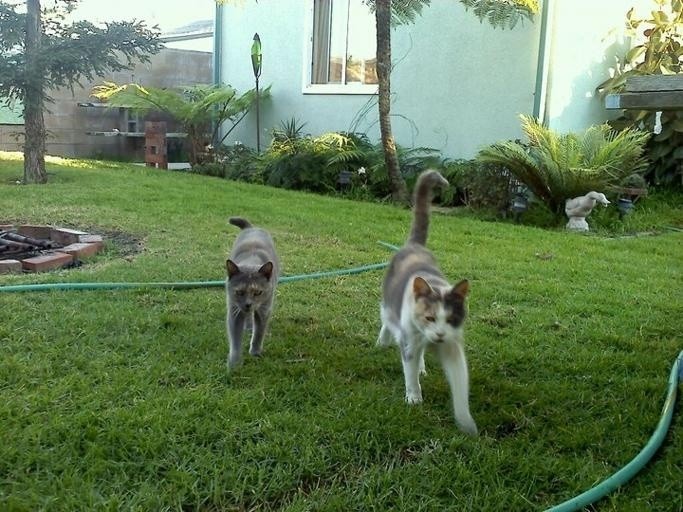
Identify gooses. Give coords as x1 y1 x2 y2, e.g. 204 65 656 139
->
564 191 611 218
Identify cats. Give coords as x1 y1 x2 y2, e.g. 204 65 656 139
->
376 168 479 437
220 215 279 373
